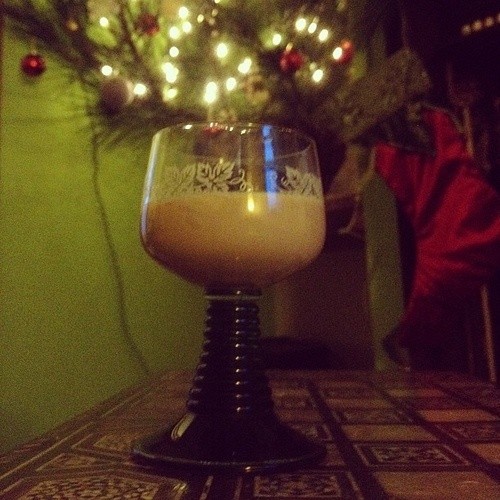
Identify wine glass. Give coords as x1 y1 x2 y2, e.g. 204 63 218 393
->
133 121 326 471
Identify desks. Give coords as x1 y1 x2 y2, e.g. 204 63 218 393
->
0 367 500 500
